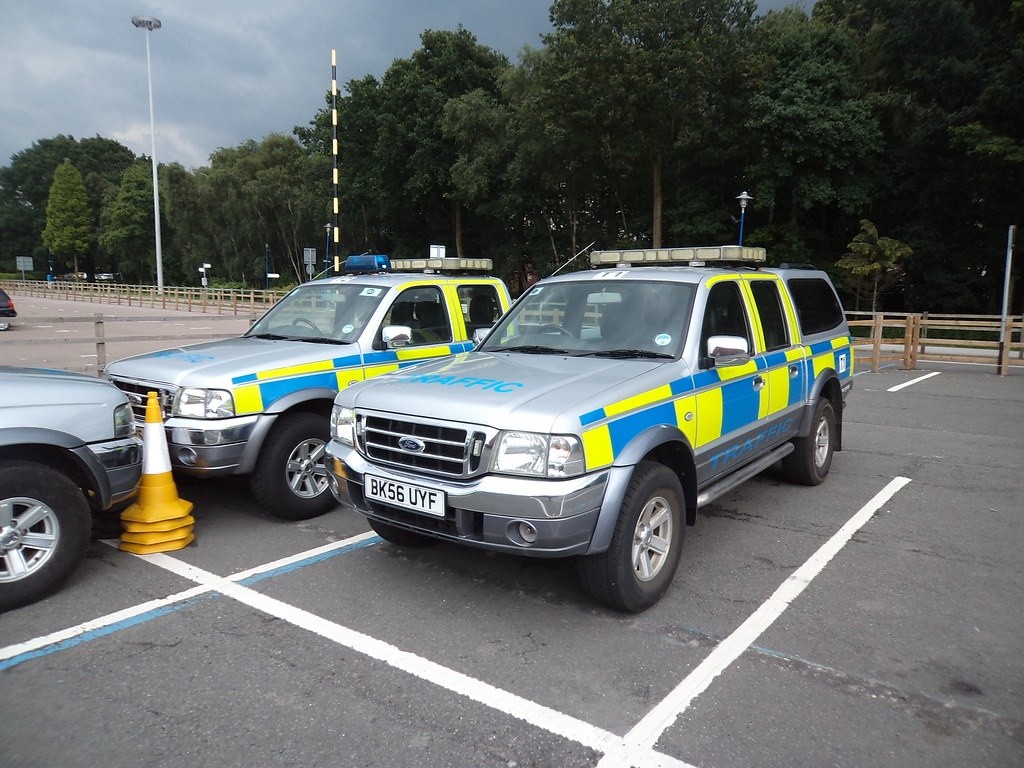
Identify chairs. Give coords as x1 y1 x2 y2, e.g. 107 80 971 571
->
602 303 718 354
470 299 493 323
390 299 445 344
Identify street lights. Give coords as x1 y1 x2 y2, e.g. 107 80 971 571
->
132 14 165 297
735 191 755 246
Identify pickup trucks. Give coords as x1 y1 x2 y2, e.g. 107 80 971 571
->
106 248 602 522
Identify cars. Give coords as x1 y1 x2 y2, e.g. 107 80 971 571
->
0 288 16 331
63 271 123 286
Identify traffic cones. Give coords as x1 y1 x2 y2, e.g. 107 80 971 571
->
119 392 197 554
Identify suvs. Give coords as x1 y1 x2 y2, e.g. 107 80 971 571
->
0 364 143 614
323 243 857 615
